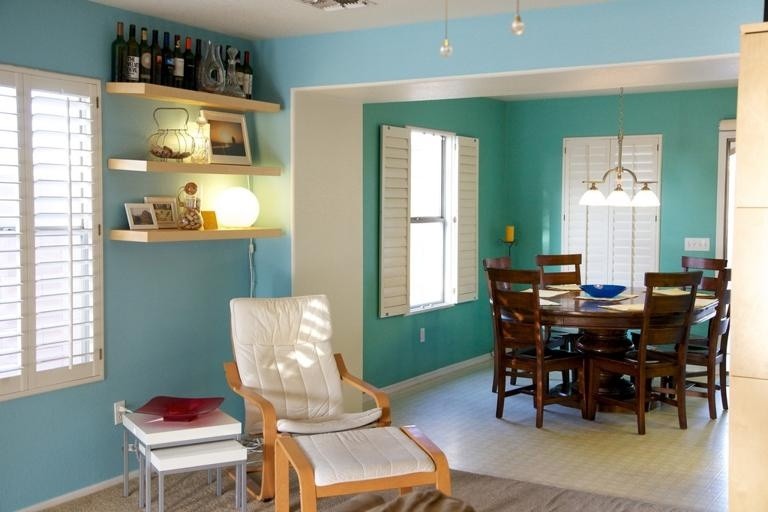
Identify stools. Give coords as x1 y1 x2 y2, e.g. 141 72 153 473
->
135 441 248 512
272 423 453 512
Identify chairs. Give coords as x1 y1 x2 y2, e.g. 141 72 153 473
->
630 256 732 420
482 254 588 429
222 291 393 504
588 271 703 434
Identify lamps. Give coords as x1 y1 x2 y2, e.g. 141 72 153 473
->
214 185 260 229
578 84 661 210
510 1 526 39
440 0 451 57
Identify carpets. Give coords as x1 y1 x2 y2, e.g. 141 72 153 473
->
39 431 707 512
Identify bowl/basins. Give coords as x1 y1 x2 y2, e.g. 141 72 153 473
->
579 284 626 298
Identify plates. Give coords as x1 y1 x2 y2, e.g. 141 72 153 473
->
131 395 225 422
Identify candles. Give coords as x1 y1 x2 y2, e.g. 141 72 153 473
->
504 225 514 243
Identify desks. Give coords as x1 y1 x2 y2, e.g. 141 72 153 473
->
521 284 719 414
116 411 252 512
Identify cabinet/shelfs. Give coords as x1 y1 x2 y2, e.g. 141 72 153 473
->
102 78 289 246
727 18 768 511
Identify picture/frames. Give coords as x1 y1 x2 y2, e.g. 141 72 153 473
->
197 110 254 167
143 196 182 228
123 202 160 232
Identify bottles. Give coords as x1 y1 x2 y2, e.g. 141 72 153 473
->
110 22 253 99
175 180 202 230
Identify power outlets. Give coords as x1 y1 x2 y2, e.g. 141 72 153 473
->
112 400 128 427
